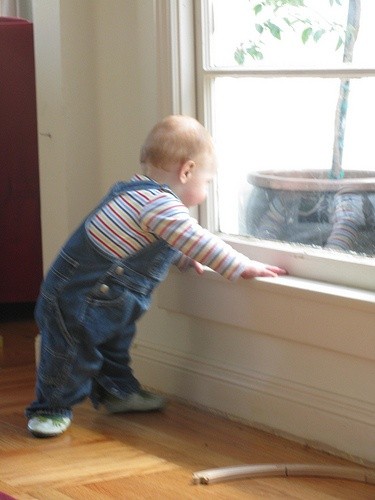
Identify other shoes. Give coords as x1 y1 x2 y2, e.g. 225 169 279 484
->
28 415 70 435
109 390 164 412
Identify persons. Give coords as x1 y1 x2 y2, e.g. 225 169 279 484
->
24 115 287 436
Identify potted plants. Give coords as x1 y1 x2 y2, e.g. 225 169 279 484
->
234 0 375 258
